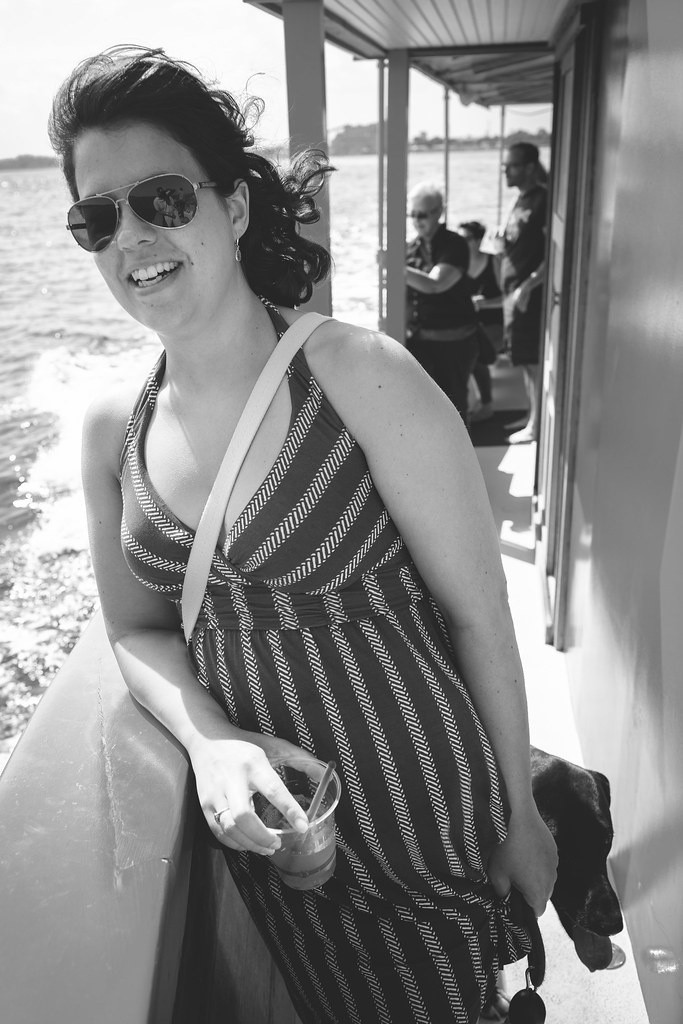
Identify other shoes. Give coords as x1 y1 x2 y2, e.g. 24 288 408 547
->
478 401 492 418
502 413 530 432
505 425 537 446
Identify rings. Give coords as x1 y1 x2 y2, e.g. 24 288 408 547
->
214 808 228 824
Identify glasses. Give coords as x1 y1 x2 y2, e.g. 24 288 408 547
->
500 162 526 173
408 205 442 219
67 174 221 254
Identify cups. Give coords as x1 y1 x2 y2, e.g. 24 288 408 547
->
250 757 344 892
471 295 485 311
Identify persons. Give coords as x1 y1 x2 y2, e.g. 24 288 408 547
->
377 142 550 445
48 44 560 1024
